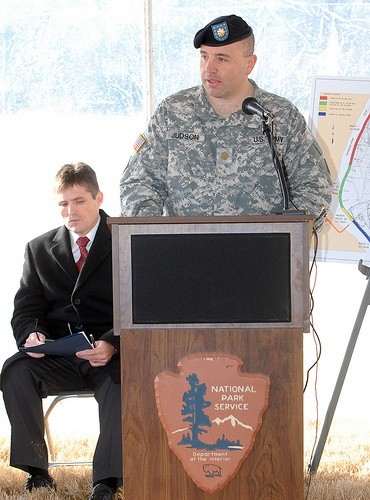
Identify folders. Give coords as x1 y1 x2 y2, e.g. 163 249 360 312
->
19 332 94 357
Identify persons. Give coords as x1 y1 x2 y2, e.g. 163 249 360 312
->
0 162 123 500
120 15 332 232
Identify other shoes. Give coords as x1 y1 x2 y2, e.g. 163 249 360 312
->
89 484 115 500
26 474 57 494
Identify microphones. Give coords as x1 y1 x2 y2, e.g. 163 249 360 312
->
242 97 274 121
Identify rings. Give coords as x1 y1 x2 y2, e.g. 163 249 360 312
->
94 360 96 363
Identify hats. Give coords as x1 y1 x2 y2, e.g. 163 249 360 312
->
194 14 252 49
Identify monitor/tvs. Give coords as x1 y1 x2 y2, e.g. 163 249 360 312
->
117 222 302 328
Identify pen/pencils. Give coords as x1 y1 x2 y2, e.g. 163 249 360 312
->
90 334 96 348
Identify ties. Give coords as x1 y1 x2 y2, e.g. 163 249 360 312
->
75 236 90 272
